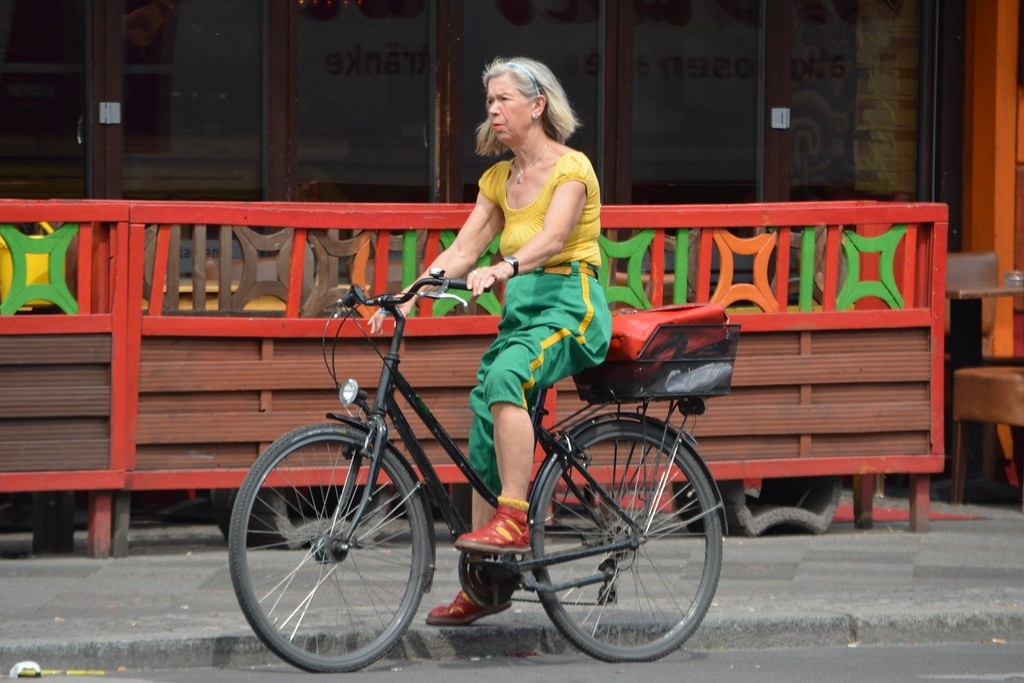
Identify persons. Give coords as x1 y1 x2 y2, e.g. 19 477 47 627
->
367 56 610 625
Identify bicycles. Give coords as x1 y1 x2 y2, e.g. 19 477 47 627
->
228 266 744 676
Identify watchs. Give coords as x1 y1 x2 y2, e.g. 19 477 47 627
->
504 256 518 278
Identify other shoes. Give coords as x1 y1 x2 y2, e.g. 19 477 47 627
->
454 498 532 553
424 570 512 627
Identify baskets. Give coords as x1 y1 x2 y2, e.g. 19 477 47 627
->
572 323 741 397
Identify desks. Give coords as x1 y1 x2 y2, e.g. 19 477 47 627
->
945 279 1024 484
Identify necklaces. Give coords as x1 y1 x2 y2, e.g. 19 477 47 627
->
517 139 550 183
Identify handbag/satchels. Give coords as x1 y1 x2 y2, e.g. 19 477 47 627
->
602 300 732 383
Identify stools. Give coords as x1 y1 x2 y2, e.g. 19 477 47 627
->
948 367 1023 506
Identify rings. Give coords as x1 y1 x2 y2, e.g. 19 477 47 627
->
473 270 478 275
380 309 389 316
491 274 497 282
